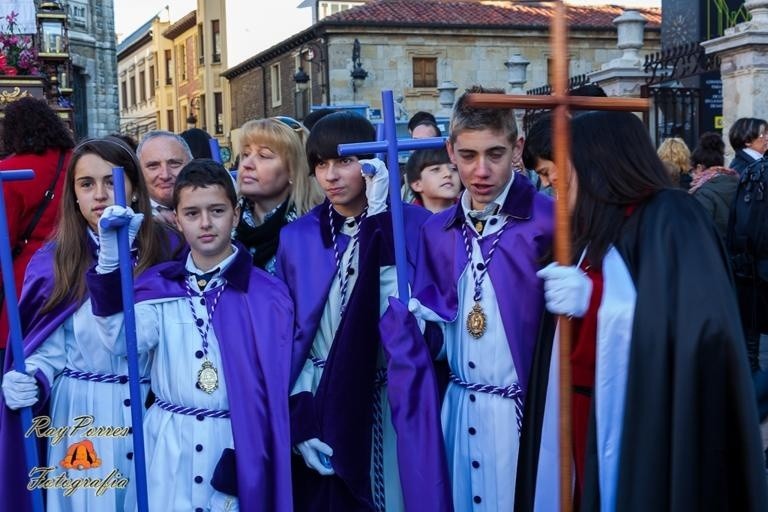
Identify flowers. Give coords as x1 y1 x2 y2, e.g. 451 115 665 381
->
1 9 39 77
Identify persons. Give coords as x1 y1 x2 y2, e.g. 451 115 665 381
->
0 97 766 426
515 105 767 512
1 136 192 511
379 85 559 511
273 111 436 511
88 159 297 512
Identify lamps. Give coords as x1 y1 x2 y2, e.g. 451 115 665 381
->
185 94 204 130
351 38 369 94
293 40 324 92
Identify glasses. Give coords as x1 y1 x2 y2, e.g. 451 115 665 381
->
753 133 768 138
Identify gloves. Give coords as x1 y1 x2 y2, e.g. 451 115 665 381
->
95 205 145 274
296 437 336 478
357 155 390 220
535 261 593 318
408 298 426 335
2 361 43 414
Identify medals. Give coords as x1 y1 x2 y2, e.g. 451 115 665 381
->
195 360 221 395
463 302 488 339
